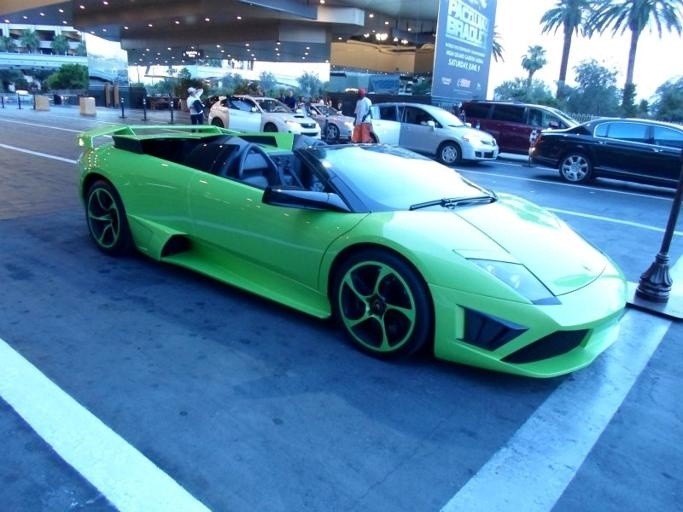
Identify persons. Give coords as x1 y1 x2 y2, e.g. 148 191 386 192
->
185 85 206 134
350 87 372 144
276 89 344 114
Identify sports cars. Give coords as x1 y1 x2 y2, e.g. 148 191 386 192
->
71 122 630 381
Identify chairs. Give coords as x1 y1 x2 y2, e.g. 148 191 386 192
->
221 138 283 188
290 135 328 188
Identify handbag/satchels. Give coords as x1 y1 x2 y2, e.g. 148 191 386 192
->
192 100 204 113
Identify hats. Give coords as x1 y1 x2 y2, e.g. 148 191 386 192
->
188 87 195 94
358 88 366 97
